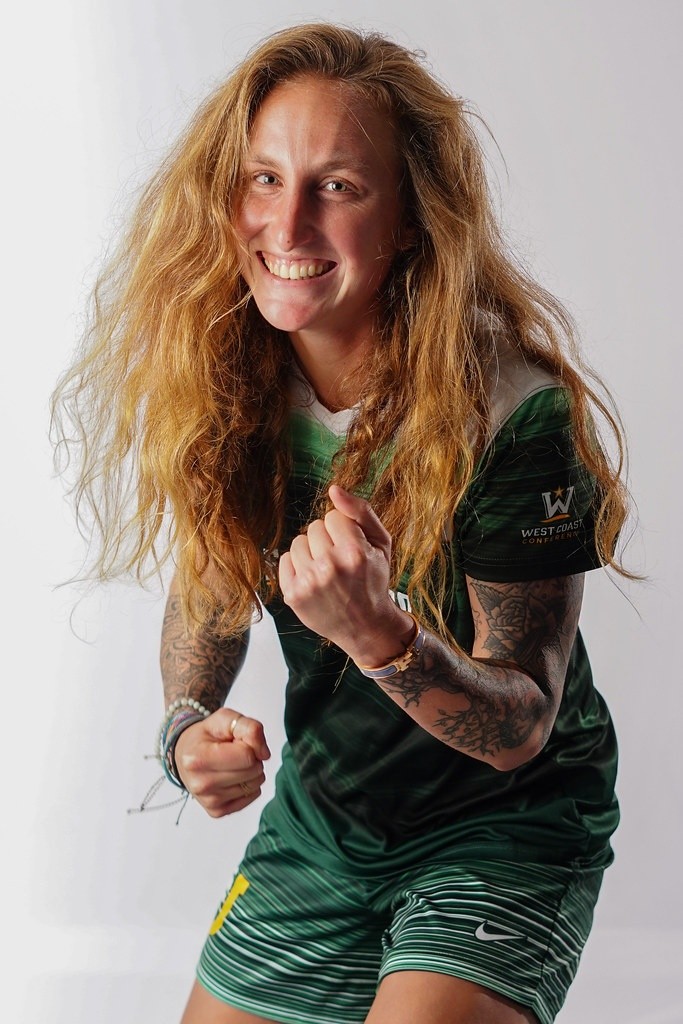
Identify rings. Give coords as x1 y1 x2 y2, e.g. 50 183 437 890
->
230 713 243 734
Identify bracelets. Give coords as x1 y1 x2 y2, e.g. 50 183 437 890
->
358 610 426 678
127 697 212 825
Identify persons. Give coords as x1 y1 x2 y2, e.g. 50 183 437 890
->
47 25 639 1024
